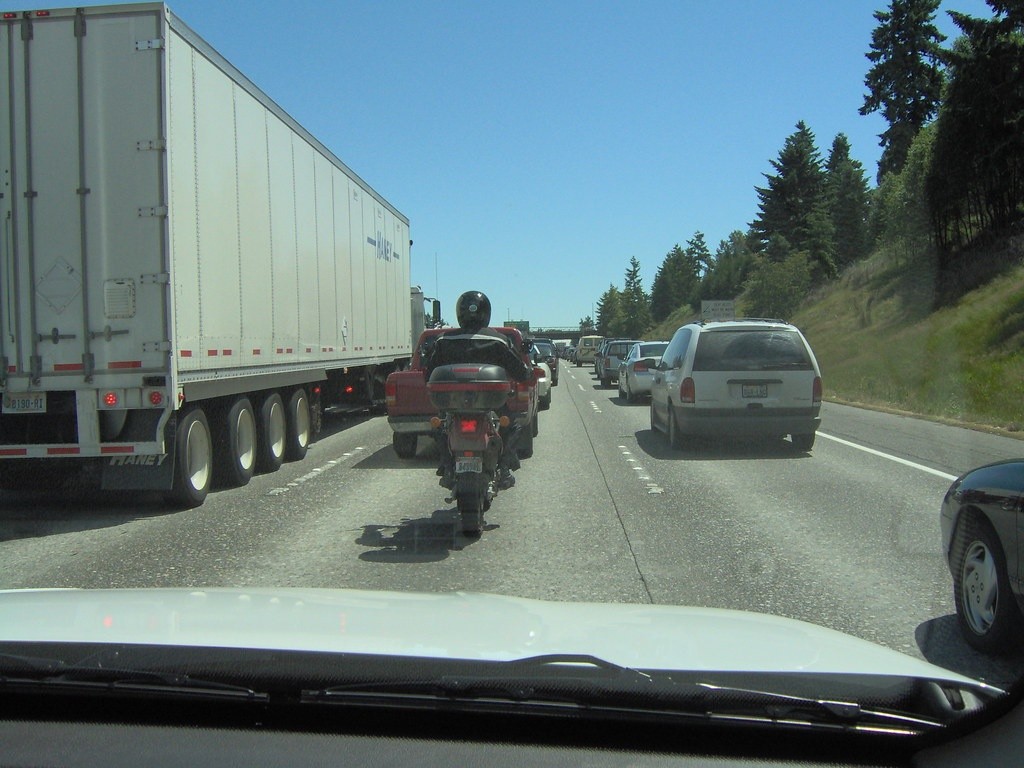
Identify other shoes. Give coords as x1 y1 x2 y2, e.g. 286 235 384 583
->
436 462 446 477
496 463 510 479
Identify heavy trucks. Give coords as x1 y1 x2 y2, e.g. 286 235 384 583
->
0 1 441 515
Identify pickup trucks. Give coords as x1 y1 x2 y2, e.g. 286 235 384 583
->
385 327 544 460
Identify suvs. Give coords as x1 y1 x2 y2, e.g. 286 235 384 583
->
576 335 604 367
649 314 823 448
525 338 561 388
594 338 646 388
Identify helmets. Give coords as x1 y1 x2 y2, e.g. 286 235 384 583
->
455 291 490 330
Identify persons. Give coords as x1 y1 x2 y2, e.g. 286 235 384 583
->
426 291 526 489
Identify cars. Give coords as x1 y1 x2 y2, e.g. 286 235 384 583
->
554 336 578 364
616 339 671 402
529 344 554 411
940 457 1023 657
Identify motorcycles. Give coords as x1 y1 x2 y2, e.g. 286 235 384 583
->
428 359 531 536
